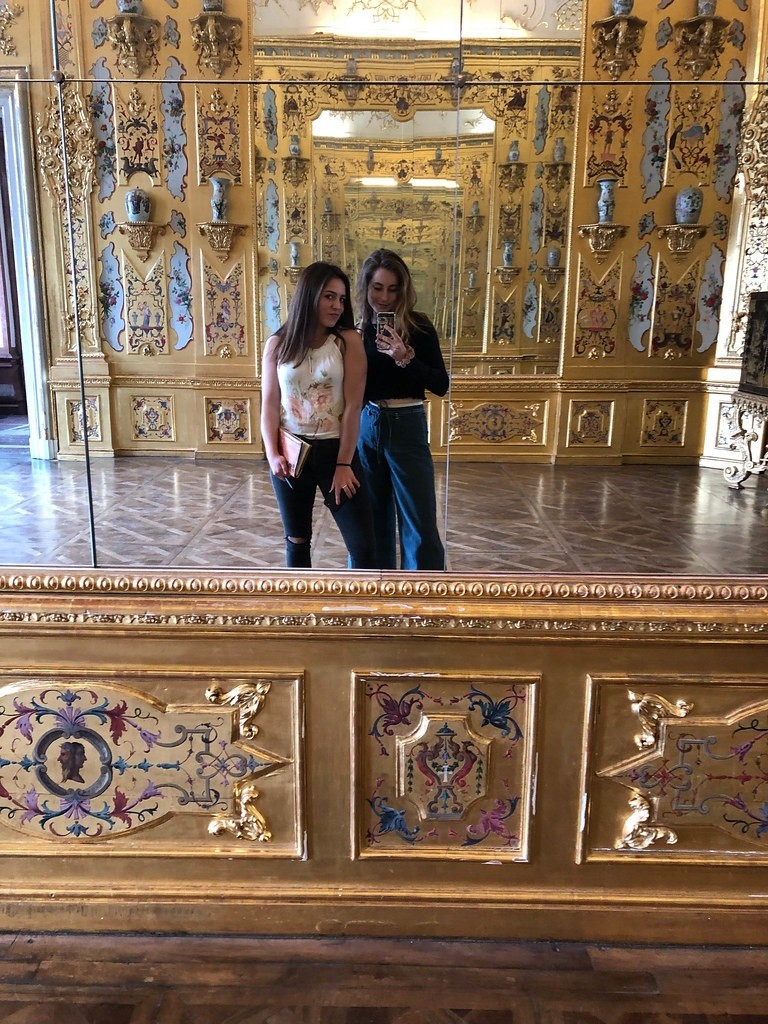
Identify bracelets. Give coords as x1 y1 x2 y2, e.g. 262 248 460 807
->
335 463 352 466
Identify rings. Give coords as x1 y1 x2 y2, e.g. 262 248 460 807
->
342 484 347 488
387 345 390 349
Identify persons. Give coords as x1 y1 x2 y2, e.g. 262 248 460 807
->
357 249 449 570
258 260 377 569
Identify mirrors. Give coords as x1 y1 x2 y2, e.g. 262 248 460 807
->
247 0 588 379
0 0 768 603
312 109 497 354
343 175 465 347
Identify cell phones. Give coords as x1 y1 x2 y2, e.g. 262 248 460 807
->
376 312 395 349
324 482 358 512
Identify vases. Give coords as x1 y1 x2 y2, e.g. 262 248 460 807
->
596 177 619 222
467 271 476 287
324 198 333 213
289 242 301 266
288 134 301 156
471 201 479 215
434 145 443 160
554 136 567 162
503 241 515 266
209 177 232 222
509 140 520 161
547 248 561 267
346 57 357 74
450 48 464 74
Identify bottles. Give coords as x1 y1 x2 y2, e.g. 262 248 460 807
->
125 186 151 221
546 244 561 267
675 183 703 224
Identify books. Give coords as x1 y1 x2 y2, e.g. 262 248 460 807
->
278 427 312 478
